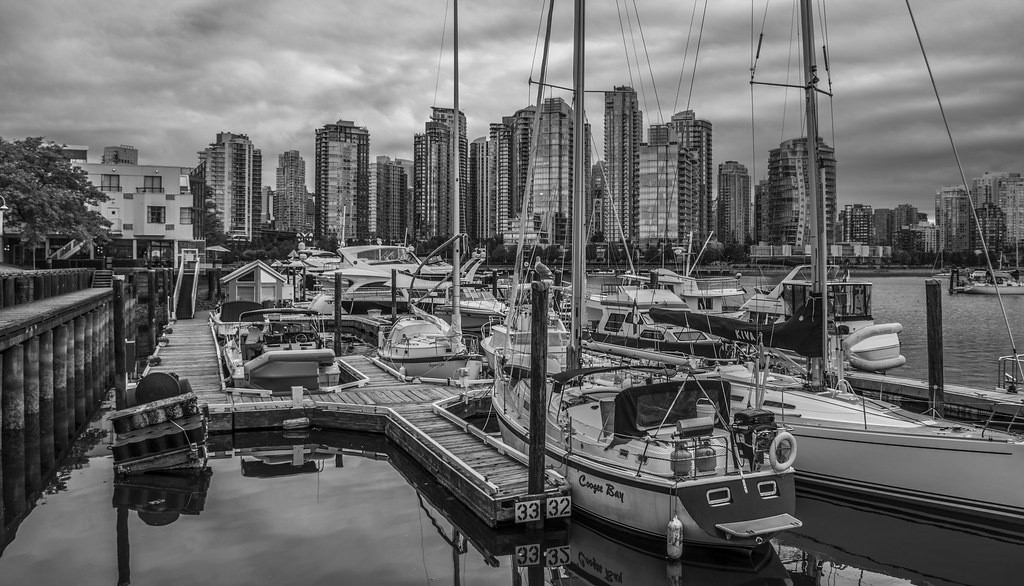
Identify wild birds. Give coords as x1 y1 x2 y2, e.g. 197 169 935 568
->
533 255 556 283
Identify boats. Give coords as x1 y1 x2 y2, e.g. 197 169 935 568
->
947 268 1024 295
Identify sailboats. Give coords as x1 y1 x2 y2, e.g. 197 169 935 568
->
209 0 1024 581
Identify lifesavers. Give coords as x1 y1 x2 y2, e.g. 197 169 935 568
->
767 430 799 474
